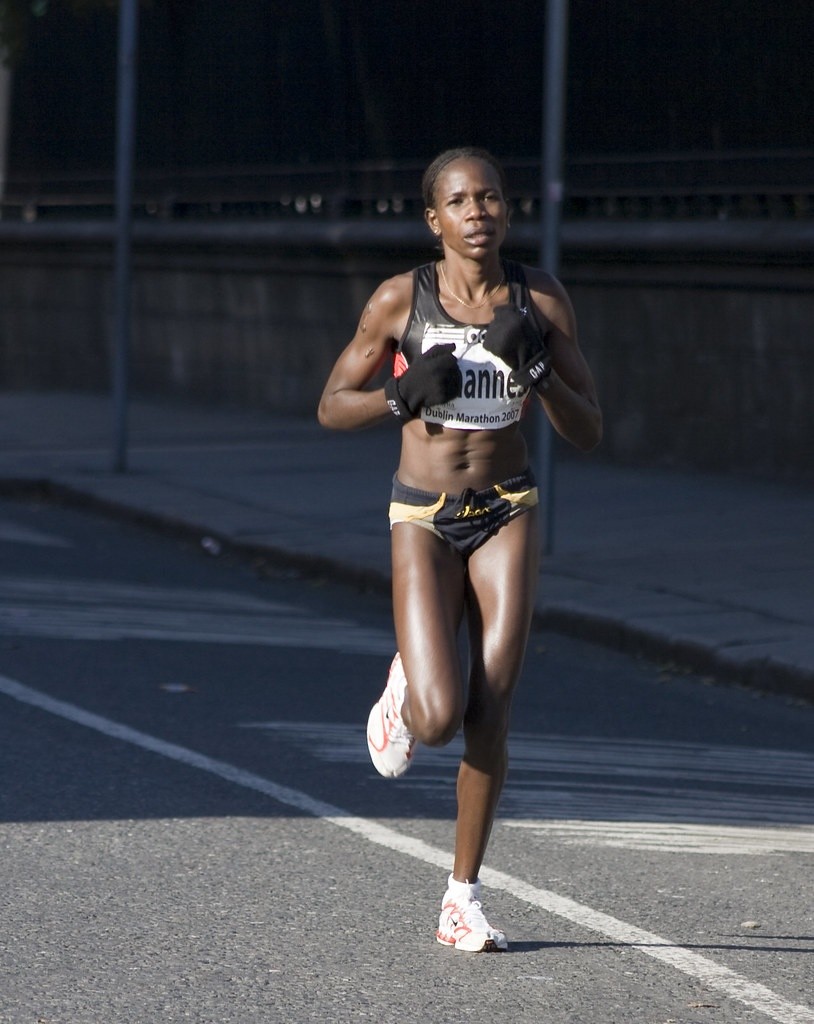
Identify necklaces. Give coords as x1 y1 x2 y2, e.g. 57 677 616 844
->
439 260 504 309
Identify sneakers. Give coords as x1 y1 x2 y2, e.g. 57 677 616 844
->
437 891 508 952
367 651 417 778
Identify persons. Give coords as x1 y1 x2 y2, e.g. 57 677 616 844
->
319 146 601 952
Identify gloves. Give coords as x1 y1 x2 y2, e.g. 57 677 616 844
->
482 304 552 388
384 343 463 425
